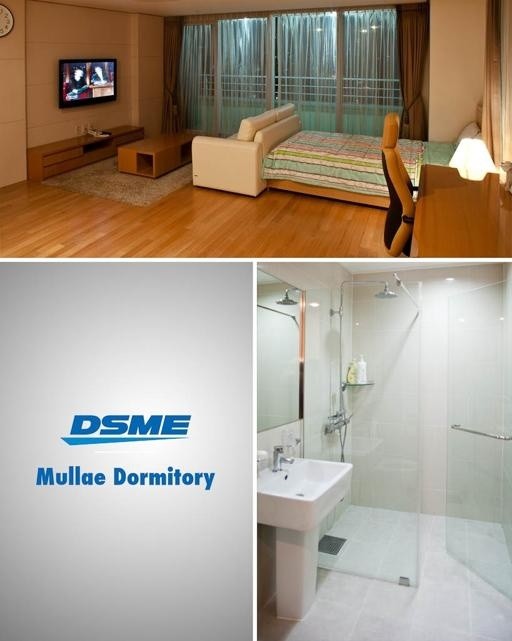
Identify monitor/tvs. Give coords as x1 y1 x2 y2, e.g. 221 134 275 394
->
59 59 117 108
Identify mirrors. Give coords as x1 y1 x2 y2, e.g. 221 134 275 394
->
257 268 305 433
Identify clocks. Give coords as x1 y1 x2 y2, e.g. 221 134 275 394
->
0 5 14 37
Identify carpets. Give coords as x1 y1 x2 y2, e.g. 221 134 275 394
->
45 154 193 206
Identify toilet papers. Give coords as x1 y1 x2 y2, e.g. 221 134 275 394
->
357 355 367 383
347 358 358 383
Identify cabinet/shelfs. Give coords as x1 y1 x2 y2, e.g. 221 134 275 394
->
27 123 145 181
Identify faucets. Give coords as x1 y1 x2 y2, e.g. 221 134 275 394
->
272 445 294 472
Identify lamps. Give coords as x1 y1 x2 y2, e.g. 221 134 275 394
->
447 135 512 192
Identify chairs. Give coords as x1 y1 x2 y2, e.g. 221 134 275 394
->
373 109 421 257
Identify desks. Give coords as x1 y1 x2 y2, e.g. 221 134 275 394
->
410 161 512 257
116 129 205 178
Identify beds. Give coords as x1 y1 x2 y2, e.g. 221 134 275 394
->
264 119 481 210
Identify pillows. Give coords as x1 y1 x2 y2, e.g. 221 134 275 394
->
454 122 480 148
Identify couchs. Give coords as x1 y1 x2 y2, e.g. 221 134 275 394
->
194 101 301 197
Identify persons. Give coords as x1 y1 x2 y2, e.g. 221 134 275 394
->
90 65 110 86
66 69 88 94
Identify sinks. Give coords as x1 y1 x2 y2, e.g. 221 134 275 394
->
257 457 352 503
